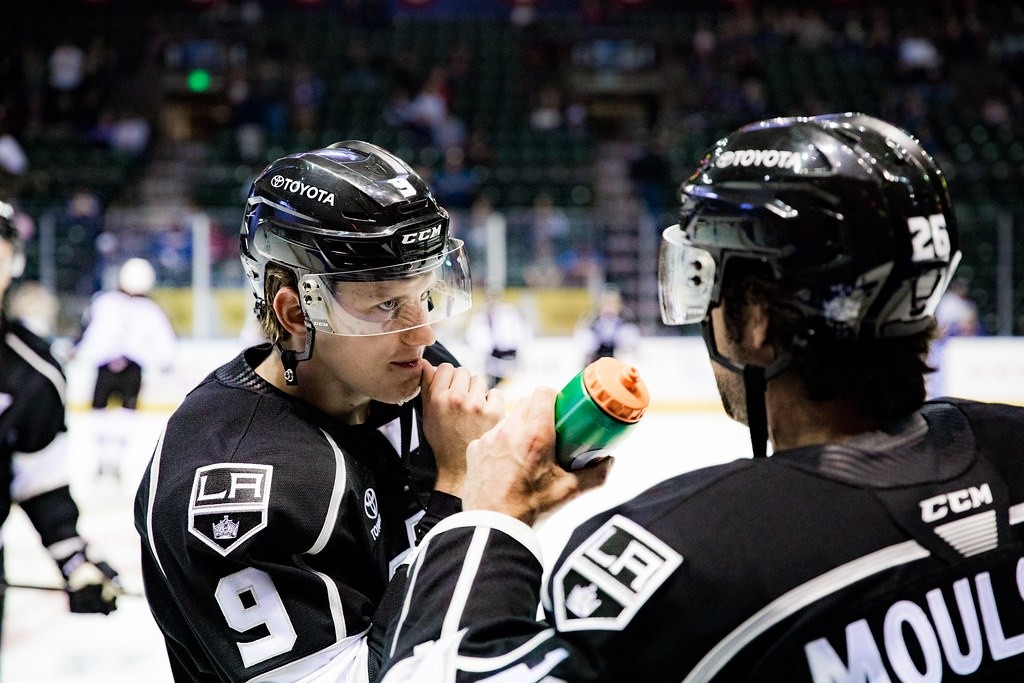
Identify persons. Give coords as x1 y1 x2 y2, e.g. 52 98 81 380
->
374 113 1024 683
0 188 125 650
133 140 506 683
2 0 1024 417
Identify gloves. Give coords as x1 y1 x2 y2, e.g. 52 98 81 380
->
54 545 124 615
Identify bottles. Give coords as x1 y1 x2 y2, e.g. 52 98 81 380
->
554 357 650 473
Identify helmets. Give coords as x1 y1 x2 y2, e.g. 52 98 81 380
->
678 110 959 345
237 139 451 331
